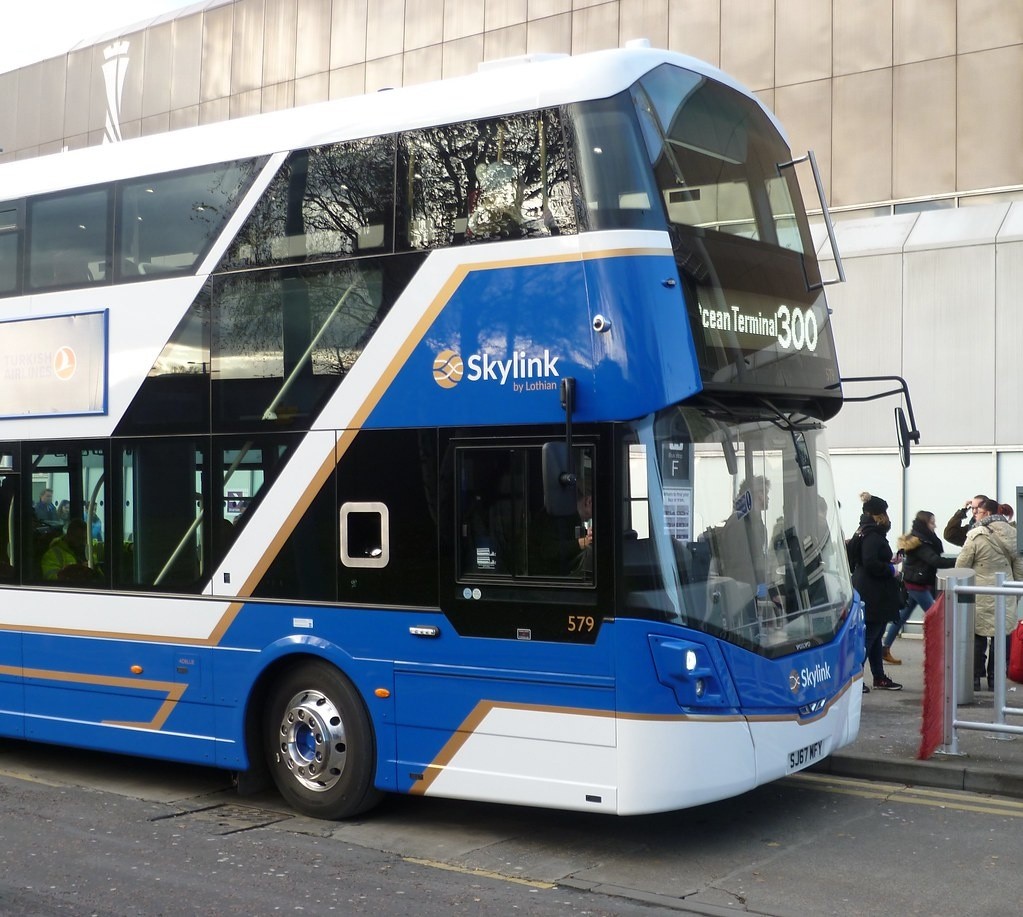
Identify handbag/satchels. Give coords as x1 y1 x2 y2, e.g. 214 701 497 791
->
896 578 909 611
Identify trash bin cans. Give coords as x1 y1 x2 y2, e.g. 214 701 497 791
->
935 567 977 705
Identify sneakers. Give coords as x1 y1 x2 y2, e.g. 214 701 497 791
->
880 645 901 666
871 675 905 693
862 683 869 693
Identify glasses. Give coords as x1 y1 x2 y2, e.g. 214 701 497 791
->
969 506 980 512
974 510 988 516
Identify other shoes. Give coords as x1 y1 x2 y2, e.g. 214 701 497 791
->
971 672 984 690
986 671 996 691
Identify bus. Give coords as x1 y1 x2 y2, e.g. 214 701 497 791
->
0 39 921 822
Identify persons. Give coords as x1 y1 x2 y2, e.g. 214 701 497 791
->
842 491 903 694
943 494 991 678
881 509 957 665
0 433 841 657
953 500 1023 693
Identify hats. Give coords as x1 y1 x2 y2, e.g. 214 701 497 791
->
860 492 889 515
